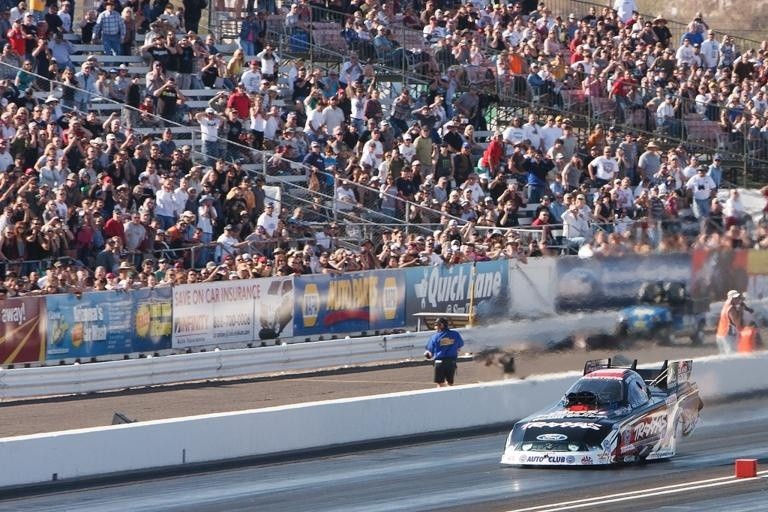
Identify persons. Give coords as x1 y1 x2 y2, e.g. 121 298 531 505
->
423 317 464 388
1 1 768 300
715 289 745 356
735 294 757 328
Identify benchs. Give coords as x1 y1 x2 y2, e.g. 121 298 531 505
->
413 312 475 333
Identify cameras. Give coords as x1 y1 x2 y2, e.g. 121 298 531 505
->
219 267 226 270
739 303 753 313
75 137 80 141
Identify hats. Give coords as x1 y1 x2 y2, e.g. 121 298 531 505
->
67 173 78 181
180 210 196 222
199 195 213 203
727 290 739 298
118 262 130 270
89 133 115 146
435 318 447 324
25 168 35 174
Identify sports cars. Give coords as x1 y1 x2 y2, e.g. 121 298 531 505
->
500 356 704 469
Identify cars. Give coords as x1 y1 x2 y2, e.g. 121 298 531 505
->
613 278 710 346
